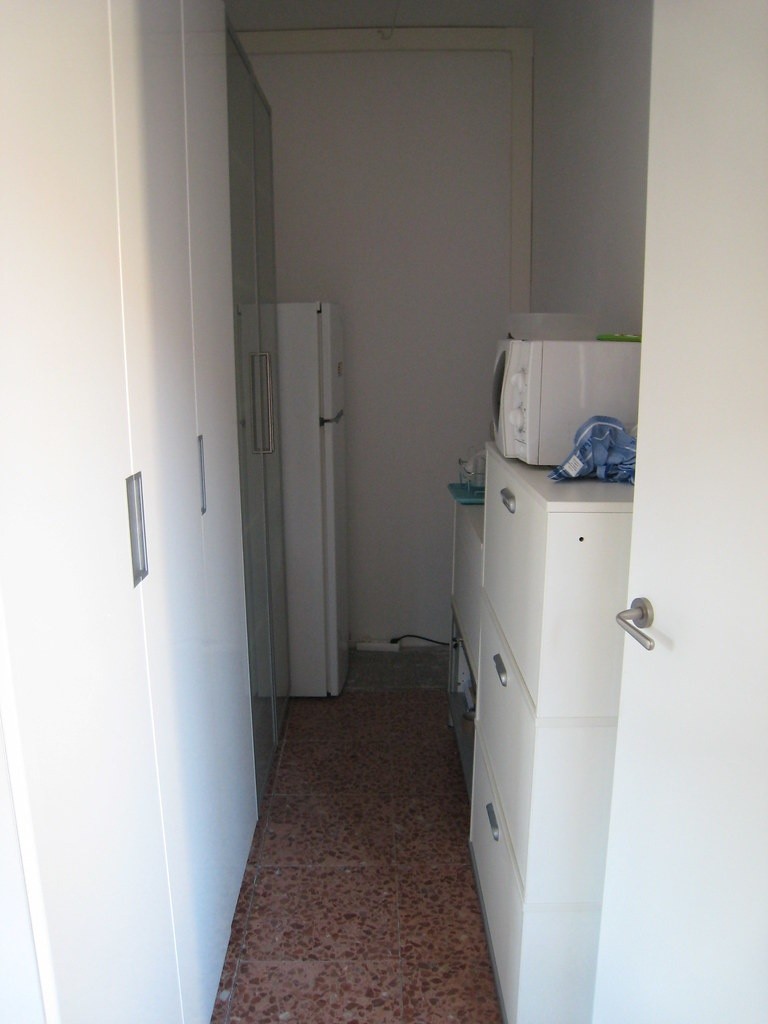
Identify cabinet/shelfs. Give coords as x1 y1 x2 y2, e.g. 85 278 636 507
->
0 0 281 1024
469 439 636 1024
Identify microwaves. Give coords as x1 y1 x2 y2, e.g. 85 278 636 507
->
489 337 642 465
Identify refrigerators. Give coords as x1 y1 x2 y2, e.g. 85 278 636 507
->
278 301 350 696
